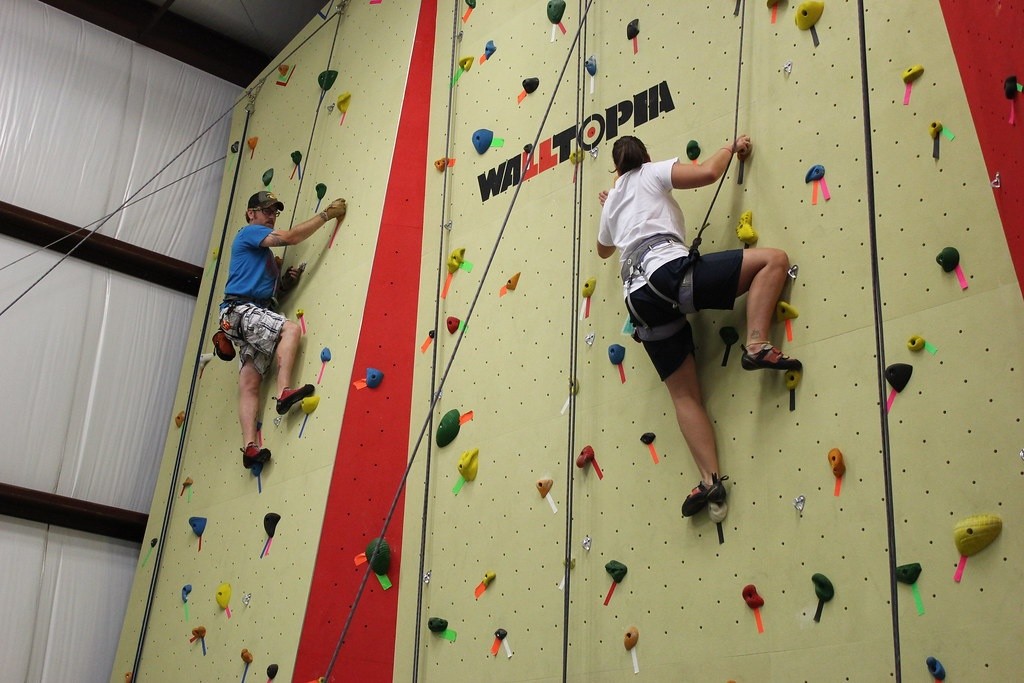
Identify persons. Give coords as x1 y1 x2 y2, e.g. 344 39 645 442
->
597 133 803 517
219 191 346 468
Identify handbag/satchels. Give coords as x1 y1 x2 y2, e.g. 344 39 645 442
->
211 331 236 361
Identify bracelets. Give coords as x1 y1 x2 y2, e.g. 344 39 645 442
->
319 212 327 221
719 147 732 153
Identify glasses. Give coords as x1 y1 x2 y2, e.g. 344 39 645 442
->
253 208 280 217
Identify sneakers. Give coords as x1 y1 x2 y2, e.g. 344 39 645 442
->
682 472 729 517
271 384 315 415
240 441 271 468
740 343 802 370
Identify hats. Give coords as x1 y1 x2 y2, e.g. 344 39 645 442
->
248 191 284 211
